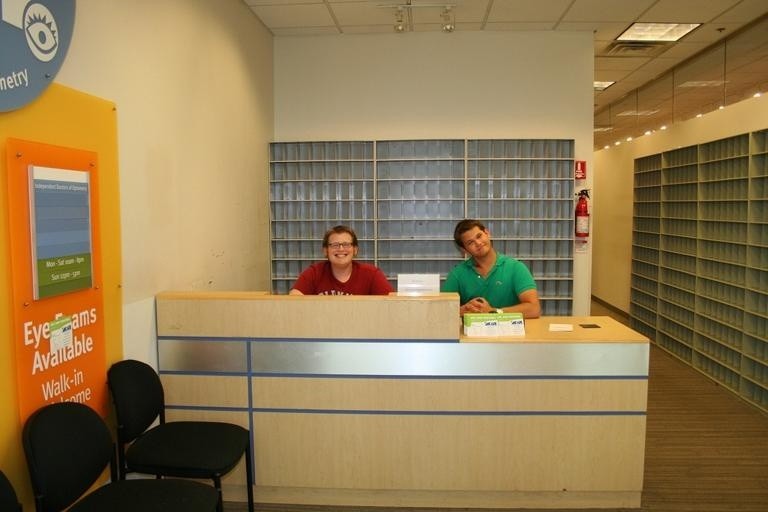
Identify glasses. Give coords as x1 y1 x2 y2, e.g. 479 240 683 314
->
327 243 353 248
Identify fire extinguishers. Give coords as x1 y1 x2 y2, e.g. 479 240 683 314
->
575 188 591 244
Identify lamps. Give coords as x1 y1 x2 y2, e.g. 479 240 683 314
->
376 4 457 33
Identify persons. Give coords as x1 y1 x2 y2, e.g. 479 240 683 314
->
440 219 540 320
288 225 397 296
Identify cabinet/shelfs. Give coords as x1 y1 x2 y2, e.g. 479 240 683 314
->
629 129 768 415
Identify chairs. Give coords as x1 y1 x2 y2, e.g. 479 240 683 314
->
0 360 255 512
